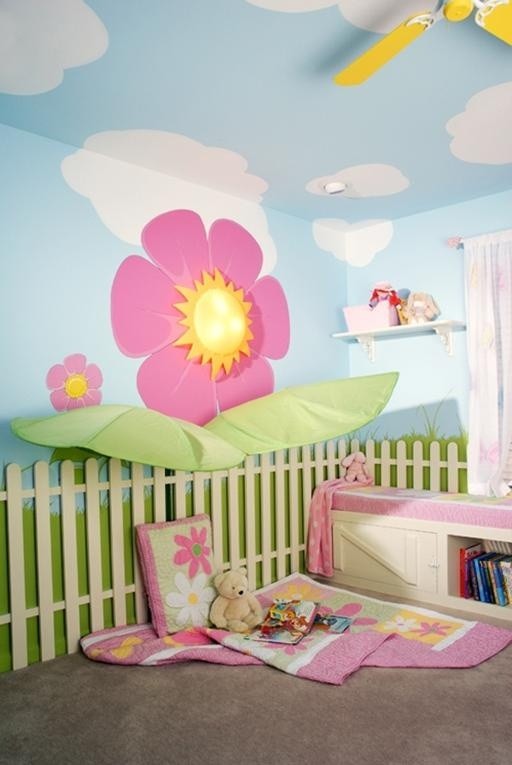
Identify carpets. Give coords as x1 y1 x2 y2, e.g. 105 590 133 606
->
76 567 512 690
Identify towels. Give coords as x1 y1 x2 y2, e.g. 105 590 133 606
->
302 474 374 580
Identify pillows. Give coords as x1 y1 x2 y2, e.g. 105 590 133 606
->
132 513 222 639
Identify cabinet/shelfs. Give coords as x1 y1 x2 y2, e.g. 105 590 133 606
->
307 478 512 631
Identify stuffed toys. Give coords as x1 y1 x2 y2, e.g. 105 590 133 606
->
367 280 402 311
207 566 262 632
397 291 440 325
341 451 373 486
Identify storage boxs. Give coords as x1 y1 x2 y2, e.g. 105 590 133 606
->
340 302 401 333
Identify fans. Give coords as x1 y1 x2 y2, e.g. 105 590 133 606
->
333 1 512 91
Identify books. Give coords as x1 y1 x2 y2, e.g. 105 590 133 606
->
243 629 304 645
316 613 356 634
458 540 512 608
260 601 321 636
